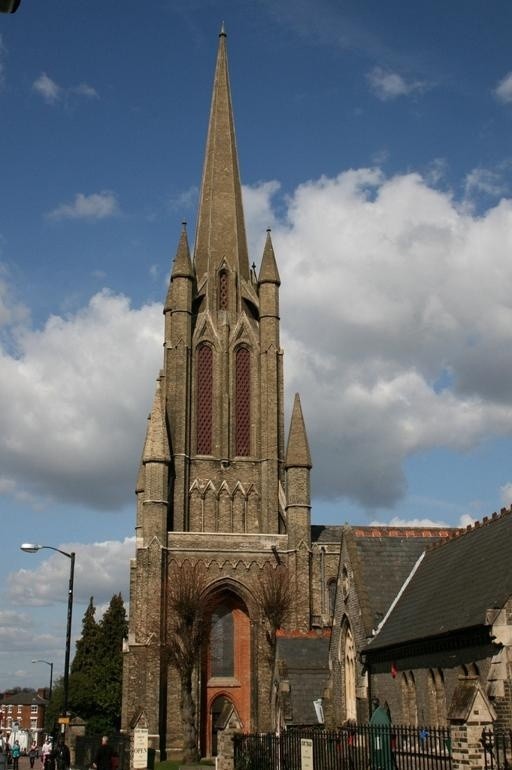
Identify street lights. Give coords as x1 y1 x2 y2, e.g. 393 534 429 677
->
20 543 75 746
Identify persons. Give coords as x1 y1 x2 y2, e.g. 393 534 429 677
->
54 738 71 770
27 741 39 769
2 746 11 770
12 740 21 770
88 735 113 770
41 737 53 769
